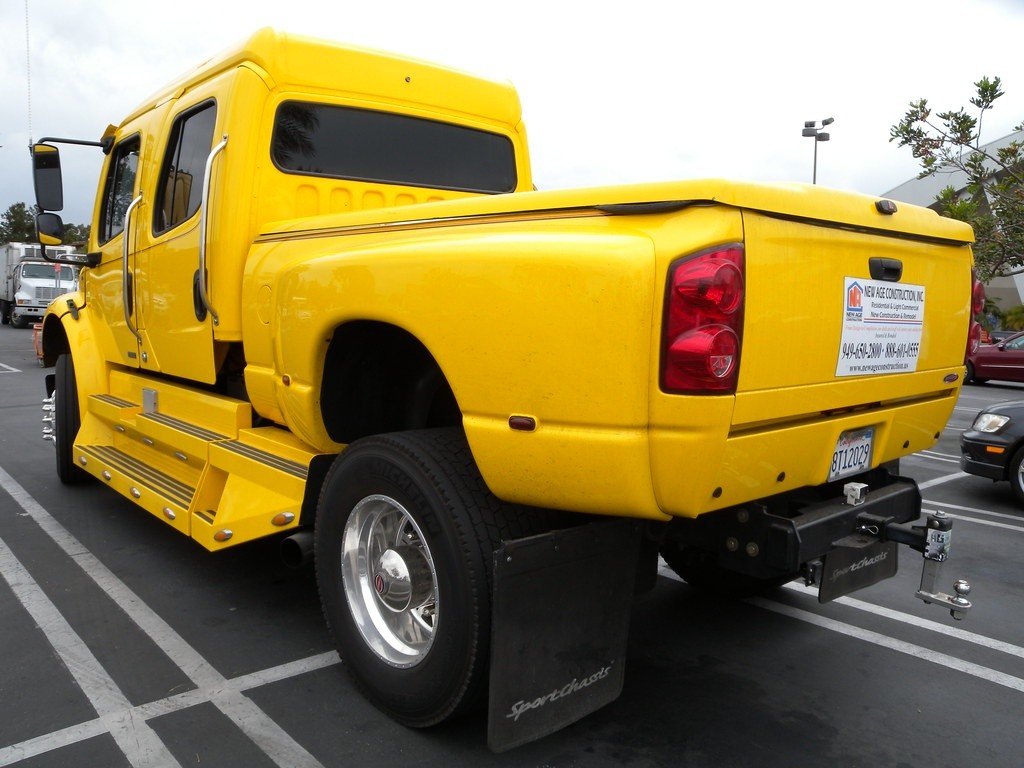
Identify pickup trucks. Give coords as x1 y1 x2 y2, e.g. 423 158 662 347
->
32 33 979 753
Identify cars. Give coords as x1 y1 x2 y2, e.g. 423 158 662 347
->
959 400 1024 506
962 330 1024 384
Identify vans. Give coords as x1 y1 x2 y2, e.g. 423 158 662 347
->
987 332 1018 346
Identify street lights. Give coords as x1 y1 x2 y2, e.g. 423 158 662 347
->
801 117 835 185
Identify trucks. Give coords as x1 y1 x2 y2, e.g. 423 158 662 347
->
0 243 78 328
